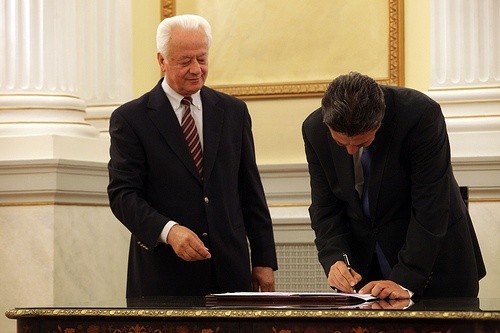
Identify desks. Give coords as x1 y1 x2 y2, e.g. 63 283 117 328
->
6 296 499 333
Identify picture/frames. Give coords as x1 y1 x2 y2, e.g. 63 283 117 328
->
158 0 407 100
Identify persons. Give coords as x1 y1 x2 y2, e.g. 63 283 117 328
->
359 301 477 311
300 72 492 301
107 14 279 311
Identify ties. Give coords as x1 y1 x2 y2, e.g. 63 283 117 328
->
181 96 205 184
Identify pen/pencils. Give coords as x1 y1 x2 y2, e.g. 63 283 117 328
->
343 252 358 294
